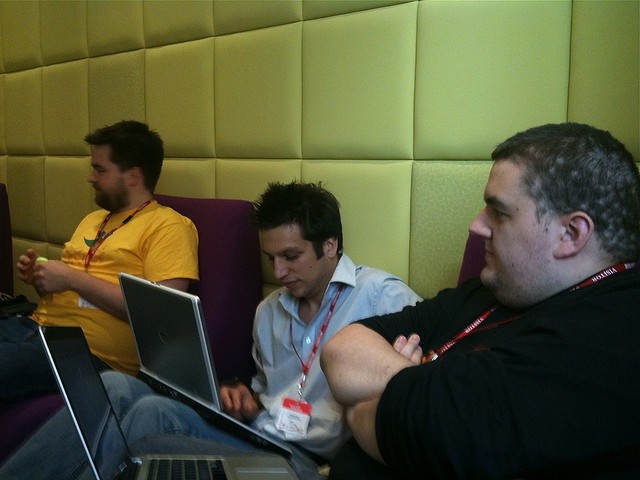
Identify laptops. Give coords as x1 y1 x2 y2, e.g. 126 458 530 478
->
117 273 293 459
38 326 299 480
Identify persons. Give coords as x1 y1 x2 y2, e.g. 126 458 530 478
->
321 124 638 478
0 180 423 480
0 121 200 404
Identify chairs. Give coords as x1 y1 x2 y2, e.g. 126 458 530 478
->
2 195 265 464
